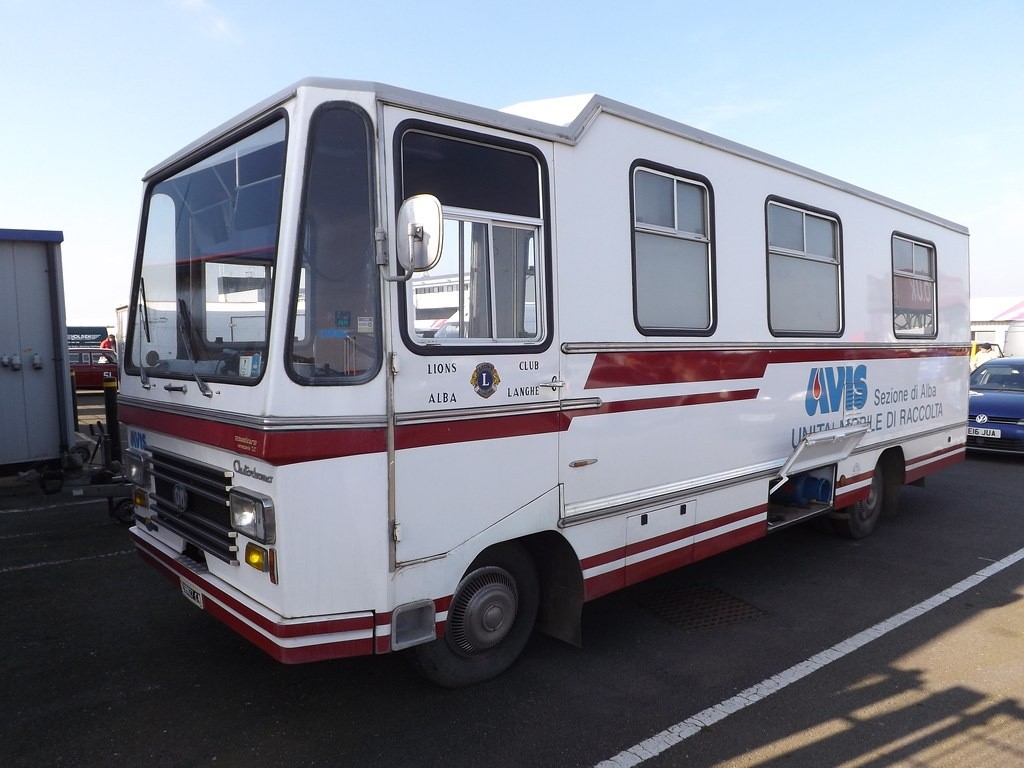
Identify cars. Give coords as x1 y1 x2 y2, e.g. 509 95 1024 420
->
966 358 1024 456
969 341 1005 373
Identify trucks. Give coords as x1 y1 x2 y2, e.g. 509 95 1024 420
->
118 77 971 695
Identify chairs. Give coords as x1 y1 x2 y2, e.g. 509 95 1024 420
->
988 374 1023 385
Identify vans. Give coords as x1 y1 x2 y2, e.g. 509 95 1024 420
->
434 301 538 338
69 347 119 390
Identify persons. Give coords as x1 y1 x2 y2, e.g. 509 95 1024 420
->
972 342 998 368
100 334 116 353
